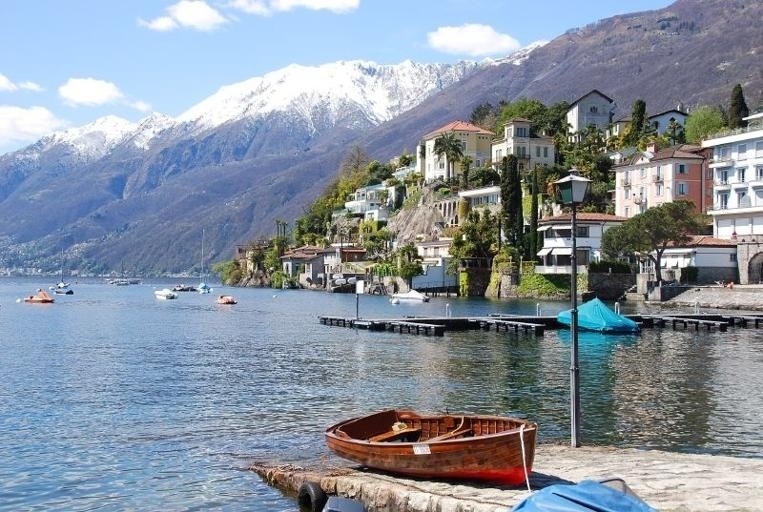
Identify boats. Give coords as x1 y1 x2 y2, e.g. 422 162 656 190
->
323 403 535 491
21 285 74 303
317 477 663 512
151 283 241 308
107 275 143 285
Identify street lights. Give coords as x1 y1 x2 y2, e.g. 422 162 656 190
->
552 167 597 452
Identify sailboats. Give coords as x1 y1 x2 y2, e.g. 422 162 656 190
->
115 258 132 287
51 248 72 290
195 228 214 294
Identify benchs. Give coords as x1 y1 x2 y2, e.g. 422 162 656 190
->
368 427 420 442
422 428 472 441
